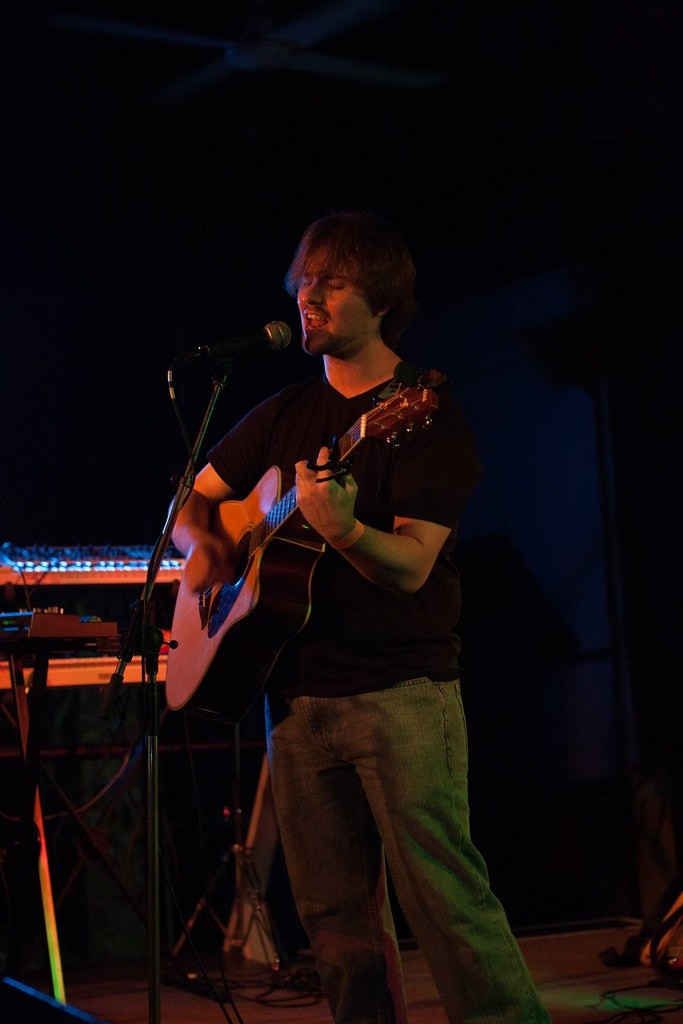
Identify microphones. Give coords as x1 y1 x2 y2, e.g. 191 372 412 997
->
193 320 292 357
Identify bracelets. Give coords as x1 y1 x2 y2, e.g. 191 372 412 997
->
330 517 364 550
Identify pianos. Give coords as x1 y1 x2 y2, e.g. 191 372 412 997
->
0 540 188 591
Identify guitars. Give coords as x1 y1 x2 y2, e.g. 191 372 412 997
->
163 372 454 717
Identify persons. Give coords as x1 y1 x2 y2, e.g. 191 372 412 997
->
168 213 551 1024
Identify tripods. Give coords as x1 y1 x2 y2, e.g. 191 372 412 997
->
166 724 291 983
